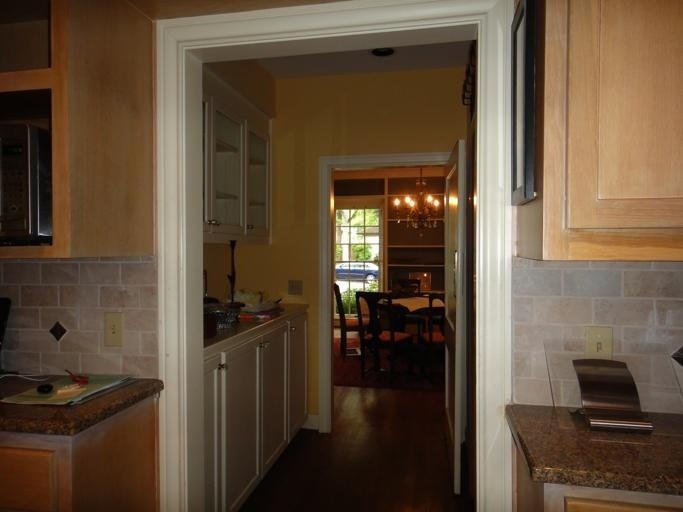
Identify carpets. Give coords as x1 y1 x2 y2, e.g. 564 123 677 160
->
331 337 444 389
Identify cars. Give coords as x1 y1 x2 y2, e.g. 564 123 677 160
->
335 262 380 283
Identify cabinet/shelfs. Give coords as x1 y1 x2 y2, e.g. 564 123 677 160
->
199 90 273 247
201 315 309 512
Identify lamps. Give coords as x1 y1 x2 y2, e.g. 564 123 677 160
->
388 168 443 233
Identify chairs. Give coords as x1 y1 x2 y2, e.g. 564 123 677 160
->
332 279 444 388
